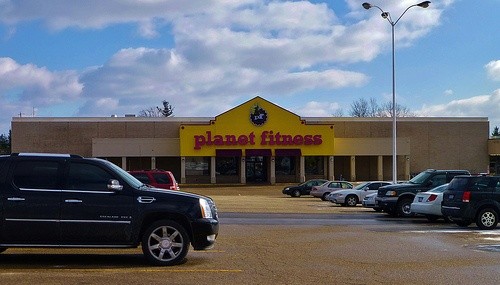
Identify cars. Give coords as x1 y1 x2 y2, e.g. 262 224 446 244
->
328 182 393 207
282 179 330 198
310 181 354 201
410 182 452 221
362 192 382 212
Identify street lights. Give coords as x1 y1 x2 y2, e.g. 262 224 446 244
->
362 1 433 187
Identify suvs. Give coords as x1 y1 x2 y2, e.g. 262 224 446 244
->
440 173 500 230
126 169 179 191
373 170 478 219
0 152 219 265
